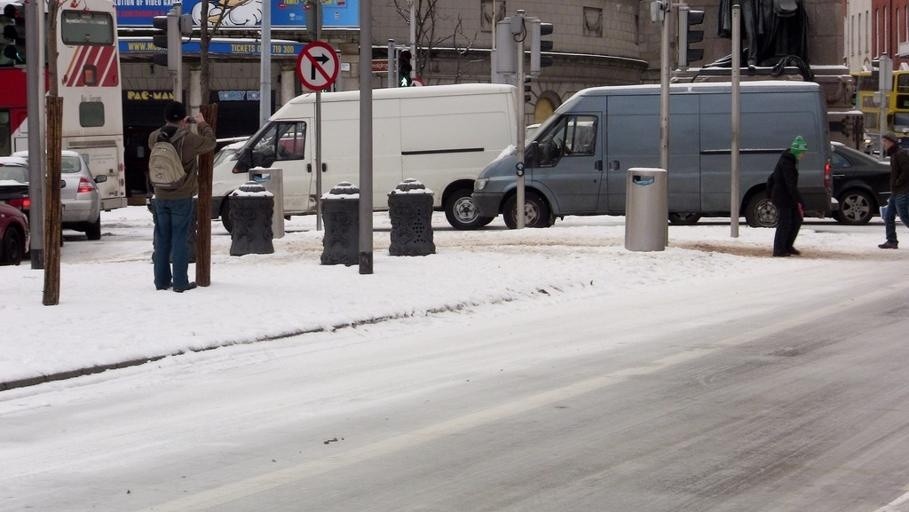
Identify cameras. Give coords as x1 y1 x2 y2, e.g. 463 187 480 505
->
187 117 195 123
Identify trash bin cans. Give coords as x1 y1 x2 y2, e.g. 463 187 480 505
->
624 167 669 252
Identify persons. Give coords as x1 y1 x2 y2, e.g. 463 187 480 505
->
768 133 808 257
878 131 909 249
148 101 216 294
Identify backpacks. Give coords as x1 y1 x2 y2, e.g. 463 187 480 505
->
149 135 196 189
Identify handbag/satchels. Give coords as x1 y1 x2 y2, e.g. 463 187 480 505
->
765 174 778 200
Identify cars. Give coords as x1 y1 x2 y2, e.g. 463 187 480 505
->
524 118 595 156
830 141 891 225
147 132 305 215
0 149 107 264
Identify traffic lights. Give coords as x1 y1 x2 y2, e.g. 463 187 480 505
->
399 50 412 86
524 77 532 102
152 16 167 66
687 10 705 61
541 22 553 68
2 3 26 65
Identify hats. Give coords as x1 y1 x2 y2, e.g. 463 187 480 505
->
790 135 808 157
166 101 185 122
882 130 898 143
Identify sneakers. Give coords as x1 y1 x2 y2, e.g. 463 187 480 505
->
174 282 196 292
786 249 800 257
157 281 173 290
879 241 897 249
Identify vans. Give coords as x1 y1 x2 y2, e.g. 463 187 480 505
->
468 82 830 227
202 81 525 227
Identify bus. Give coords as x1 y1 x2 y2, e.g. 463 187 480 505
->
852 69 909 140
0 0 128 211
